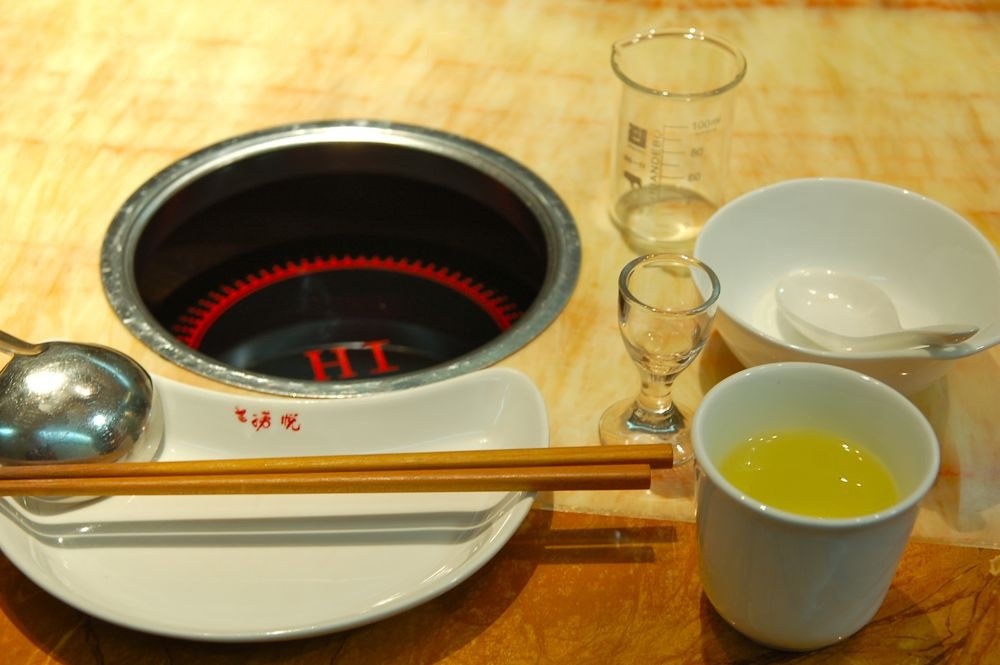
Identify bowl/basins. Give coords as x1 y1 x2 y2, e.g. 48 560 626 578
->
690 175 1000 395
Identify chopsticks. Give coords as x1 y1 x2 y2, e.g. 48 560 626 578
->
1 443 675 497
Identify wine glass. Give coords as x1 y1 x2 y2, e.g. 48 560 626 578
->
598 252 726 468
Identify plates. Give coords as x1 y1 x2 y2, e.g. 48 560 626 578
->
0 366 549 642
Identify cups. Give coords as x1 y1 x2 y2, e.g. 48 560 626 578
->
689 360 940 651
606 29 748 246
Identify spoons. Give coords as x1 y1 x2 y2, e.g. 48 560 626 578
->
779 265 979 352
0 329 164 505
